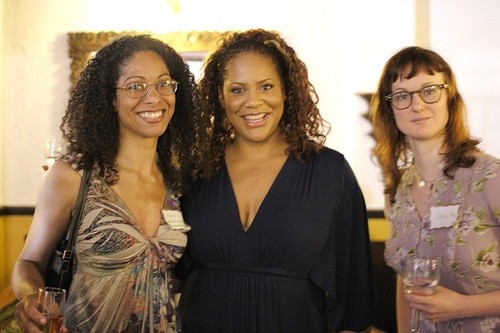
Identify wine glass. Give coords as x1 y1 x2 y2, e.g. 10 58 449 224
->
402 257 437 333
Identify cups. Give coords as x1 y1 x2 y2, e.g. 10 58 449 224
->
39 287 66 333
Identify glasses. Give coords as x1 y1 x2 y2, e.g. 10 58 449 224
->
114 79 179 98
384 83 448 110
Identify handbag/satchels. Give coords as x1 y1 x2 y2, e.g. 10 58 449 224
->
42 160 93 306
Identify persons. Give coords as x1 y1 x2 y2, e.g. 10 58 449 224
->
11 35 199 333
172 27 379 333
371 47 500 333
